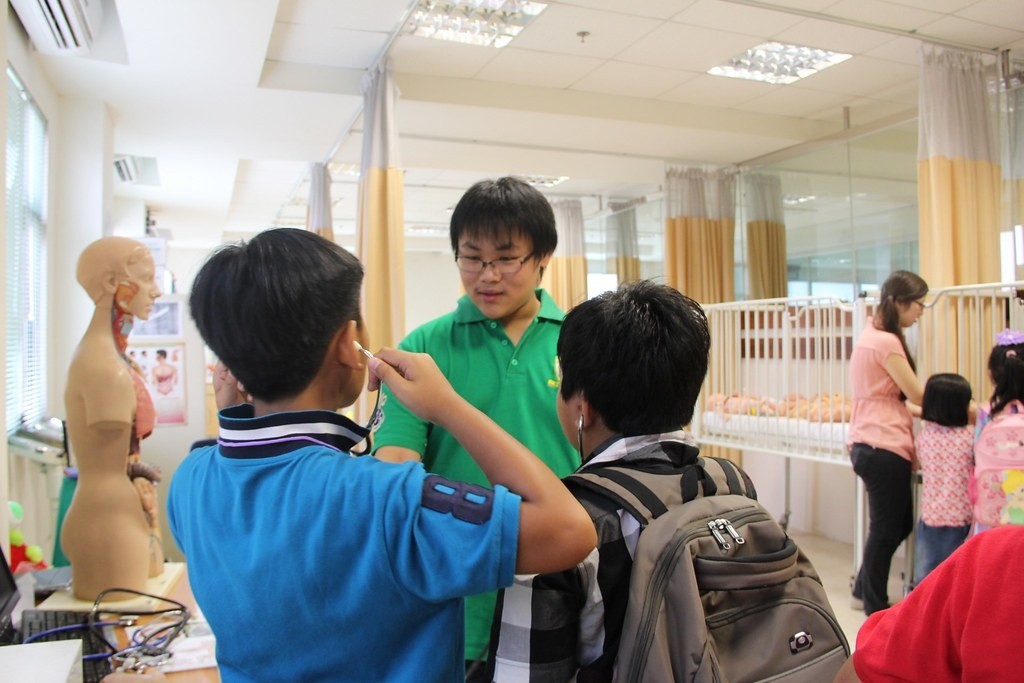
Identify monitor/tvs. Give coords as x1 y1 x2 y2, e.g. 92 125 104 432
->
0 547 22 647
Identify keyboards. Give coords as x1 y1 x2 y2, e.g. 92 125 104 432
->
21 609 112 683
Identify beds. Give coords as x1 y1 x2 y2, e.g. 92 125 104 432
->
689 295 880 588
901 280 1023 596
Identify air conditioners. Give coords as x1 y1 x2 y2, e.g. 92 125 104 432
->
8 0 103 54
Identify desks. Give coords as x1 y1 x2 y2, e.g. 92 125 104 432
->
39 564 224 682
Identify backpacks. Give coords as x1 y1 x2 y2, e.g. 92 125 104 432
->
968 405 1022 527
560 453 853 683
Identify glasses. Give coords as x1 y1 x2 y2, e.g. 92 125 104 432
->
911 300 927 307
454 248 539 274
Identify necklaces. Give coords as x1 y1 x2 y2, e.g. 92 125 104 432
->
217 436 340 450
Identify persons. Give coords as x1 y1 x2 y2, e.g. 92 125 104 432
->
830 524 1024 683
845 269 926 617
706 391 850 423
167 228 599 683
491 280 757 683
972 328 1024 537
59 235 165 602
372 177 586 683
915 374 975 589
